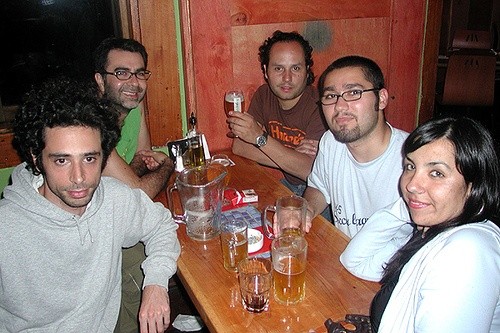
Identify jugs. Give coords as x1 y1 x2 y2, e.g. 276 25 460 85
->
166 164 228 242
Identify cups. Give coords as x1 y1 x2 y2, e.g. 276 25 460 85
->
263 194 307 240
235 257 273 313
219 220 248 273
271 236 309 304
211 153 235 187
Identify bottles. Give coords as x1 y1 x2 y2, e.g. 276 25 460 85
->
185 112 208 184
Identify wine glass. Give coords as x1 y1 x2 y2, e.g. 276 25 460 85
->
224 88 245 138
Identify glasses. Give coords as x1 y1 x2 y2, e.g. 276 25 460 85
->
105 70 152 80
319 88 381 105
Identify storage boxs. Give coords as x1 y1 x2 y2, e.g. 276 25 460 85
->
227 189 258 206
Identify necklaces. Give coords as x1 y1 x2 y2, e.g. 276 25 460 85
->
118 138 133 161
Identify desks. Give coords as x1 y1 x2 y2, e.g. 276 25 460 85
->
152 148 382 333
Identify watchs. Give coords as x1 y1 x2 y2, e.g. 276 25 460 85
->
254 130 270 148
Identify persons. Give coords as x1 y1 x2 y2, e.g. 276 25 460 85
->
272 55 411 241
94 38 177 200
0 77 181 333
225 29 334 226
369 116 500 333
